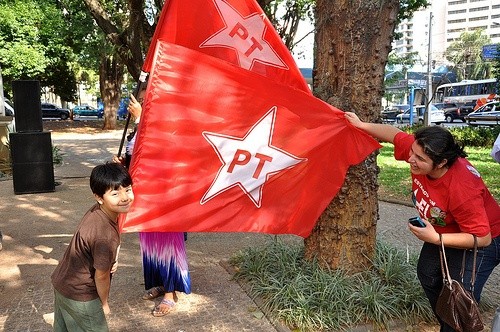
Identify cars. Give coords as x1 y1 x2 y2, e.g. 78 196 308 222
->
464 100 500 127
72 104 103 117
380 103 447 125
41 103 70 120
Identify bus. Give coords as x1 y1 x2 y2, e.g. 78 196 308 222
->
434 78 500 123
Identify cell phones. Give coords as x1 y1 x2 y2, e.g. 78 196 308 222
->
408 217 426 228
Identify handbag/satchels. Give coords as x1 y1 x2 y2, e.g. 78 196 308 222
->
436 232 484 332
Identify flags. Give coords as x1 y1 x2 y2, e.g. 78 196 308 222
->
139 0 312 96
118 41 383 240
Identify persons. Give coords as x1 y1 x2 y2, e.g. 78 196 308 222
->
50 163 135 332
113 81 190 317
0 229 2 250
345 111 500 332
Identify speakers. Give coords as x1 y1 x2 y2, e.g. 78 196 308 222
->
11 79 42 132
8 130 56 194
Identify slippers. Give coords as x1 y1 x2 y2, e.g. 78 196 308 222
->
143 287 168 300
152 296 178 316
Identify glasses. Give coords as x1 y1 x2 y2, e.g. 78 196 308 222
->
138 98 145 103
